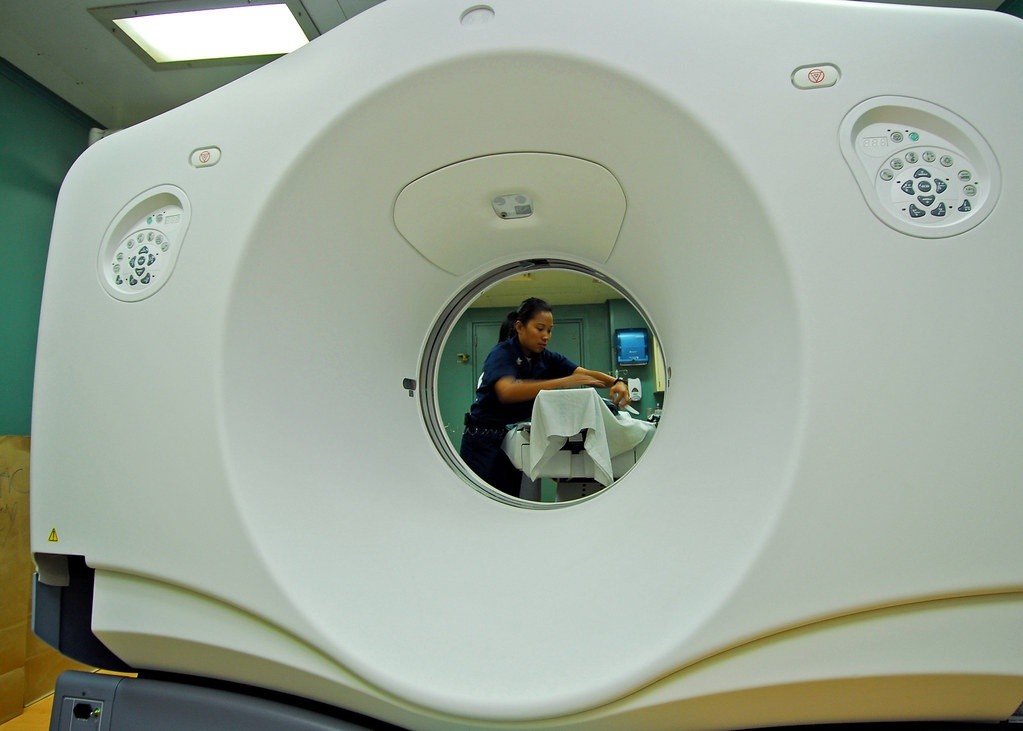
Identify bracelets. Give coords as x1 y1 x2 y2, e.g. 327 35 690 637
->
613 377 628 385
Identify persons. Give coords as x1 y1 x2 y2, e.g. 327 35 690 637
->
460 297 630 498
